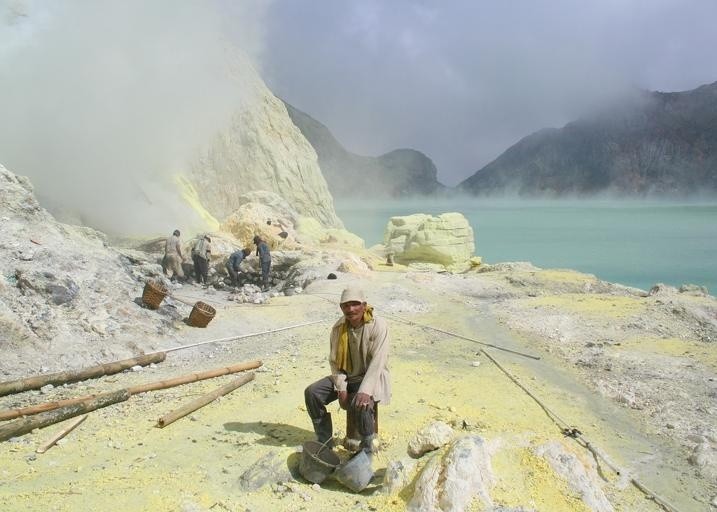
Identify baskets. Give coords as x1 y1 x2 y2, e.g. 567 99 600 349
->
188 301 216 328
142 280 168 309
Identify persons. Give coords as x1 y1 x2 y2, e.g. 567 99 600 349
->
304 288 392 453
191 235 211 285
225 247 251 287
253 236 272 292
164 229 187 282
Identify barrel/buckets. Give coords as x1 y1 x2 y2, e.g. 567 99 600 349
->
299 440 340 484
335 449 372 495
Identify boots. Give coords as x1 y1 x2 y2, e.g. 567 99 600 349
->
311 412 335 449
359 433 374 464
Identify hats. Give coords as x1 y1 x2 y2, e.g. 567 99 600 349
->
174 230 180 236
204 235 211 242
340 287 365 307
254 236 261 244
244 248 251 255
